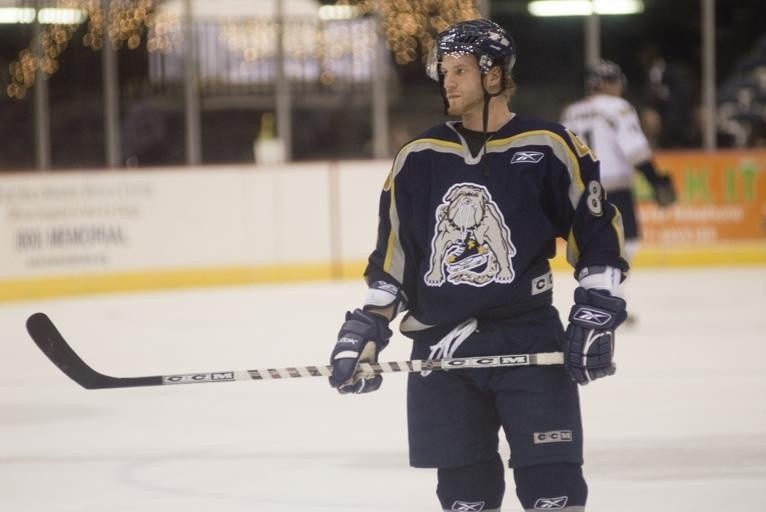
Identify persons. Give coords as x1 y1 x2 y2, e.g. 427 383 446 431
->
558 56 680 328
323 17 630 512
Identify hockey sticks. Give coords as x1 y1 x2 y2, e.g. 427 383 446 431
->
24 312 562 388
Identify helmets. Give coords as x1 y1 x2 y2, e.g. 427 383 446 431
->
435 18 519 81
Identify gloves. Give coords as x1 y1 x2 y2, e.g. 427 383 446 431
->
325 306 395 398
561 287 631 386
